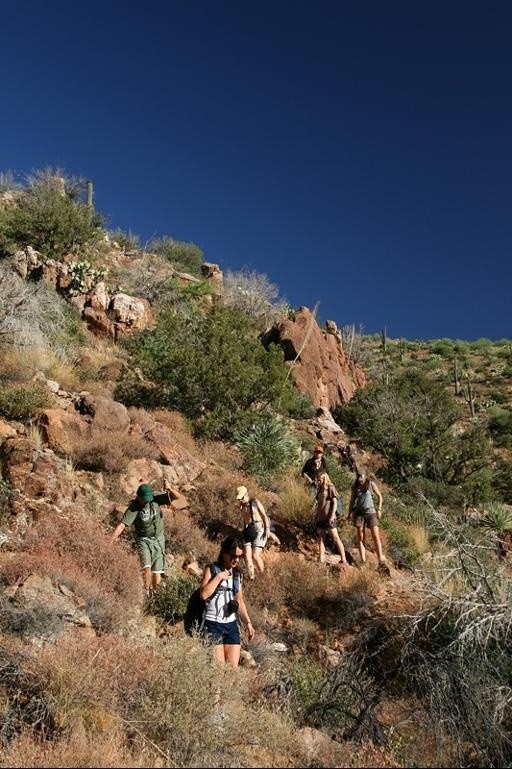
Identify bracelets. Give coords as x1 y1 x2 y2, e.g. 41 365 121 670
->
377 506 382 511
265 526 269 528
245 622 252 625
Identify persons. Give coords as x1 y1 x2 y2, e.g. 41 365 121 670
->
302 446 329 490
344 468 388 564
199 535 256 722
108 477 180 598
311 471 348 566
231 484 273 580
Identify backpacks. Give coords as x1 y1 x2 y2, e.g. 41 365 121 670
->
183 562 218 638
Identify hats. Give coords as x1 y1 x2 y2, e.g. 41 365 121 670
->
136 483 155 502
236 485 248 500
313 445 324 454
355 469 368 481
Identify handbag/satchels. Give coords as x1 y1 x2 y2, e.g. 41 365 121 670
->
335 494 345 516
241 523 258 543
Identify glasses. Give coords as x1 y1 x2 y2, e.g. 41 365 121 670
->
228 552 245 560
